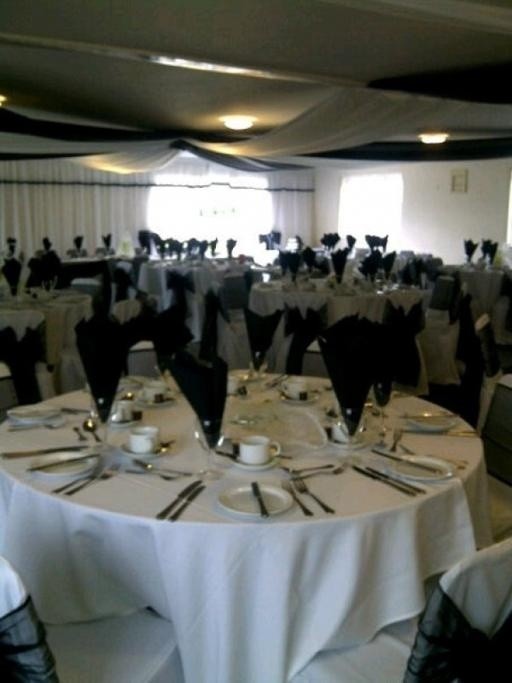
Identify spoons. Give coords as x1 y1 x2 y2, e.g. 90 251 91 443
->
83 417 102 442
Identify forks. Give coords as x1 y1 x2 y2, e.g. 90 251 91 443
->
66 463 121 496
54 459 107 493
74 426 88 442
293 477 336 514
291 462 348 481
281 481 316 516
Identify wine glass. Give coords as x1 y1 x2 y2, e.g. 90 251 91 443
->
365 270 395 295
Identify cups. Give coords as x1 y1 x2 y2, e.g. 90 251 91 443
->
129 425 160 453
112 400 133 420
281 376 309 399
331 419 365 443
138 381 168 402
239 435 281 464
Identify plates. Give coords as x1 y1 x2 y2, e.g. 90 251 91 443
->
6 404 69 427
135 397 176 409
217 484 295 519
231 453 280 471
386 414 456 434
31 450 97 476
389 455 456 483
108 419 139 429
118 441 171 457
326 436 369 450
279 393 321 406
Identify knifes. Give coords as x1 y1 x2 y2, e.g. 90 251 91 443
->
155 480 203 520
169 484 206 521
251 481 269 518
366 467 425 494
354 467 417 497
